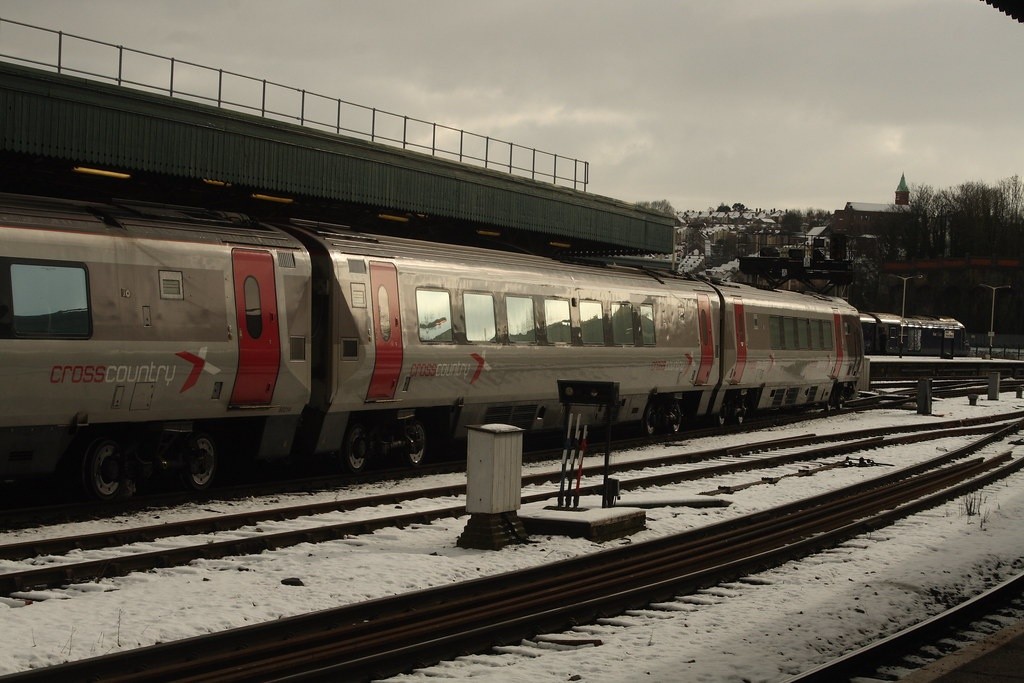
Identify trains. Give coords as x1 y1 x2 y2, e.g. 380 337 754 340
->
0 187 871 514
858 312 971 356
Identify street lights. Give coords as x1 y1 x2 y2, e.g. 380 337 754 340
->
979 283 1012 360
887 272 923 358
